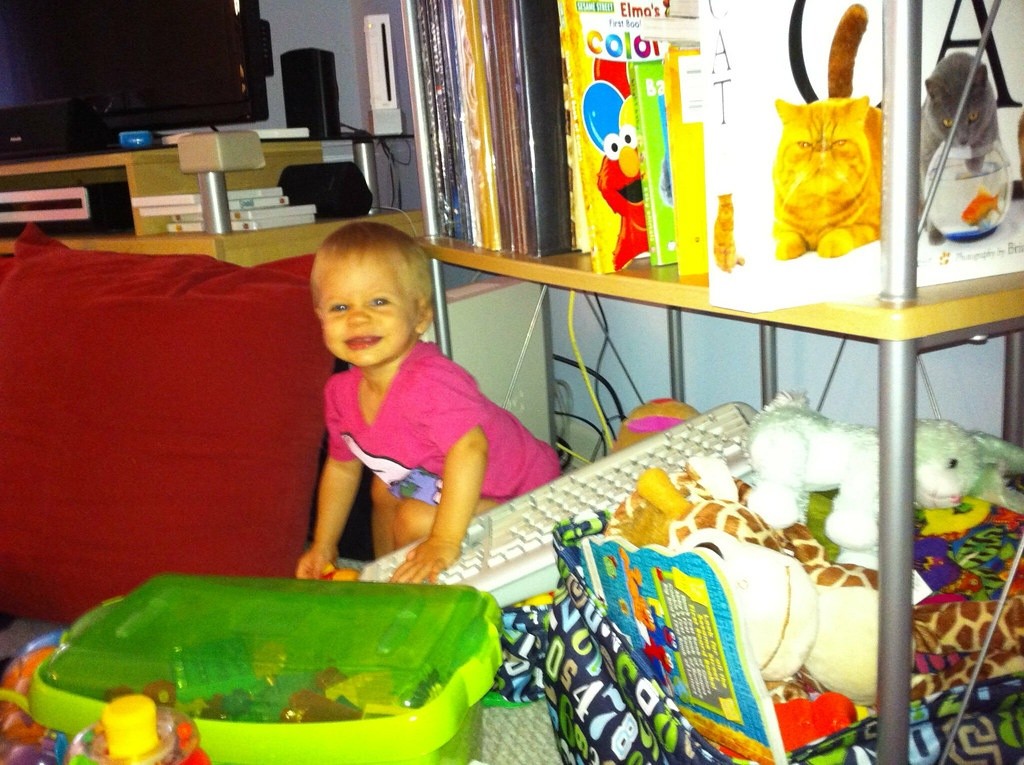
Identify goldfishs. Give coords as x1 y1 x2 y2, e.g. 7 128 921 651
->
960 186 1002 225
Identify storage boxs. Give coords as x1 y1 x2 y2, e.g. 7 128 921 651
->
697 0 1024 314
28 573 505 765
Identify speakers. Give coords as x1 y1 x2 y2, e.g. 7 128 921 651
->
84 181 135 235
278 161 373 219
281 46 342 142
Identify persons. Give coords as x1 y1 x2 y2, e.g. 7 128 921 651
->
294 221 560 585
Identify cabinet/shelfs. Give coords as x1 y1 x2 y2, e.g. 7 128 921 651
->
347 255 558 454
399 0 1024 765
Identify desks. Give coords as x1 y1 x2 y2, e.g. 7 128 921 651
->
0 132 435 238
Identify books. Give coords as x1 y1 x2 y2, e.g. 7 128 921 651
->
414 0 1024 313
581 534 788 765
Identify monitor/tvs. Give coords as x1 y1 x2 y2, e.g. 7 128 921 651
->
0 0 270 162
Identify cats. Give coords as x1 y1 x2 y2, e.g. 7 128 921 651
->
773 3 885 259
915 52 1000 245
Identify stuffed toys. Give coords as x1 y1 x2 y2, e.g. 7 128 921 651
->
601 391 1024 711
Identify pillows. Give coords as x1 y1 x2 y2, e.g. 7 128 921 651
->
0 217 340 627
